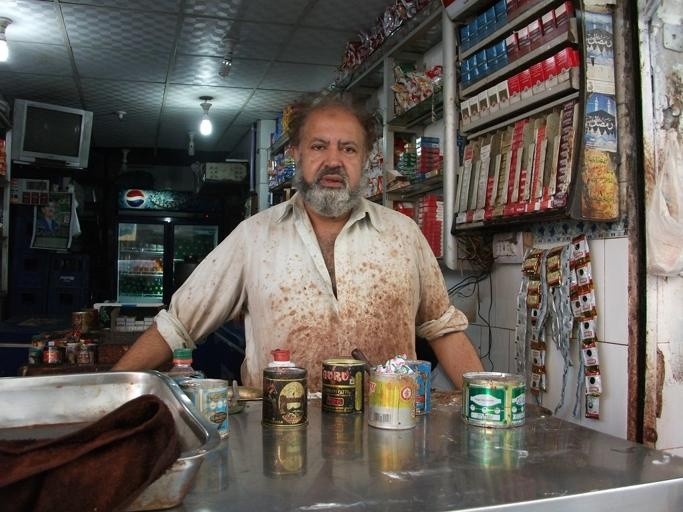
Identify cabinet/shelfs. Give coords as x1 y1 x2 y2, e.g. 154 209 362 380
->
268 0 621 273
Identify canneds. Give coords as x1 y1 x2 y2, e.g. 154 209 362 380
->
187 444 230 495
368 355 432 430
261 428 308 481
26 307 99 368
180 377 229 442
369 415 431 481
321 415 364 461
461 423 529 471
321 357 364 415
462 370 526 428
261 368 306 426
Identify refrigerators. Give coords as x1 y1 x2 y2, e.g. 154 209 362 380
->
116 188 226 305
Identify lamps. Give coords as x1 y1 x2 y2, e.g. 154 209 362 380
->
199 95 214 135
116 111 127 120
187 132 196 156
0 16 14 62
217 38 238 80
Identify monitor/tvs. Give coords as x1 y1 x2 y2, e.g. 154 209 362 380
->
11 99 94 170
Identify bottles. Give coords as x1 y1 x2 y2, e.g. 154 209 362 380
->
267 348 296 368
168 347 194 376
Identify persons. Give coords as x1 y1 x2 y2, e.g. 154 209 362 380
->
36 200 61 238
107 92 486 393
